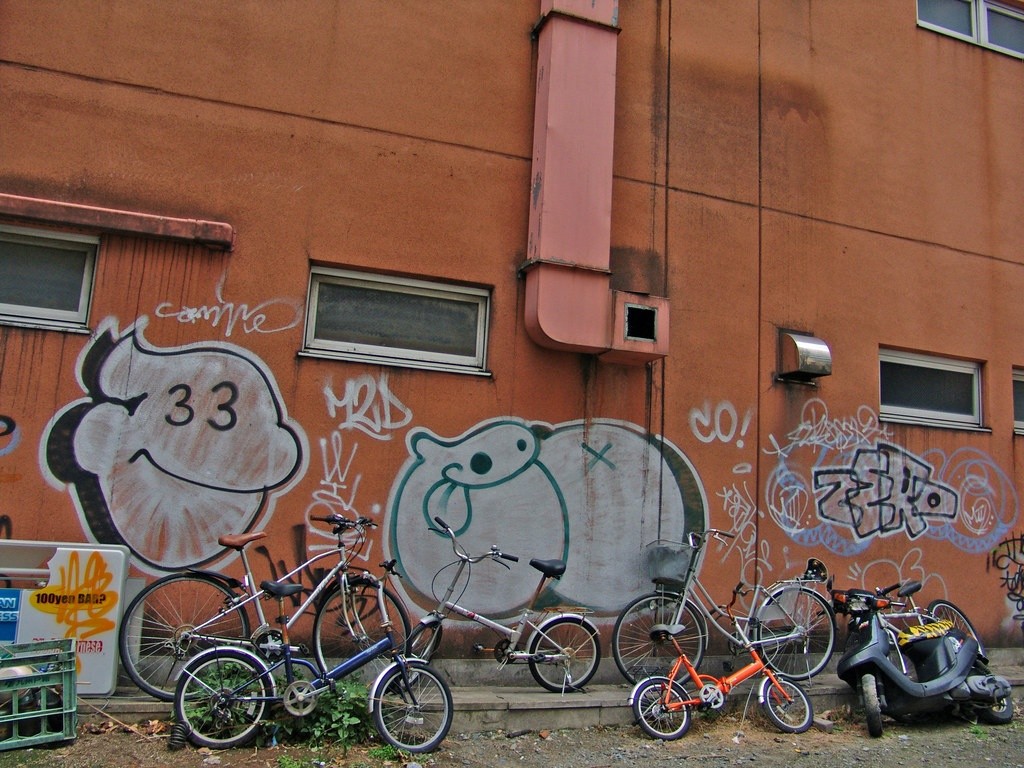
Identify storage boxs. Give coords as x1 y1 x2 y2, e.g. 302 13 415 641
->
0 637 78 750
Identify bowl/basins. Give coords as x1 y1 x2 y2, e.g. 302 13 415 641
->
0 665 33 704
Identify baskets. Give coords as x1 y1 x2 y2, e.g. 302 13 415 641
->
646 539 693 583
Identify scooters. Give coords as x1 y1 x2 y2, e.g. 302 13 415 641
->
817 576 1014 738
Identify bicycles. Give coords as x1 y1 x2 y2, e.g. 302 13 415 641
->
613 529 837 692
829 580 987 682
394 516 602 696
118 514 411 705
628 580 814 740
173 556 453 753
